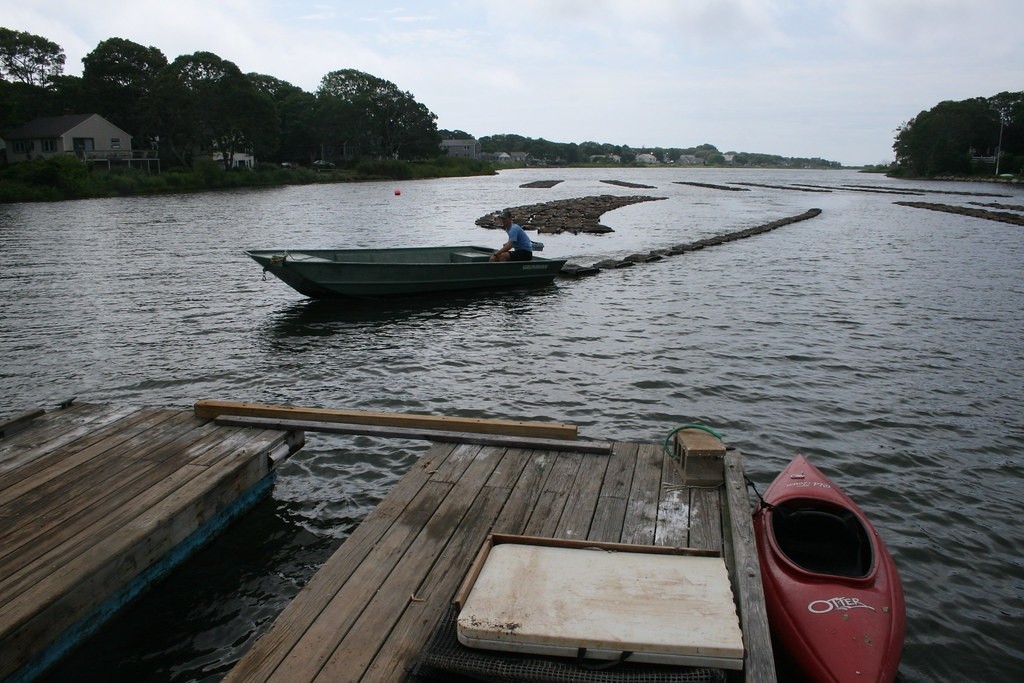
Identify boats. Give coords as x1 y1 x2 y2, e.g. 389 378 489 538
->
243 246 568 298
753 454 906 683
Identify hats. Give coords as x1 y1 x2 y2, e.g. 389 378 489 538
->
498 210 511 219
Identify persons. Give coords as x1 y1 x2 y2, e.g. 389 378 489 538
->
489 210 532 262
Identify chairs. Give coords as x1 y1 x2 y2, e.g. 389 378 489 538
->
782 509 848 567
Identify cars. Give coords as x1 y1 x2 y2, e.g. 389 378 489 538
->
314 160 334 170
